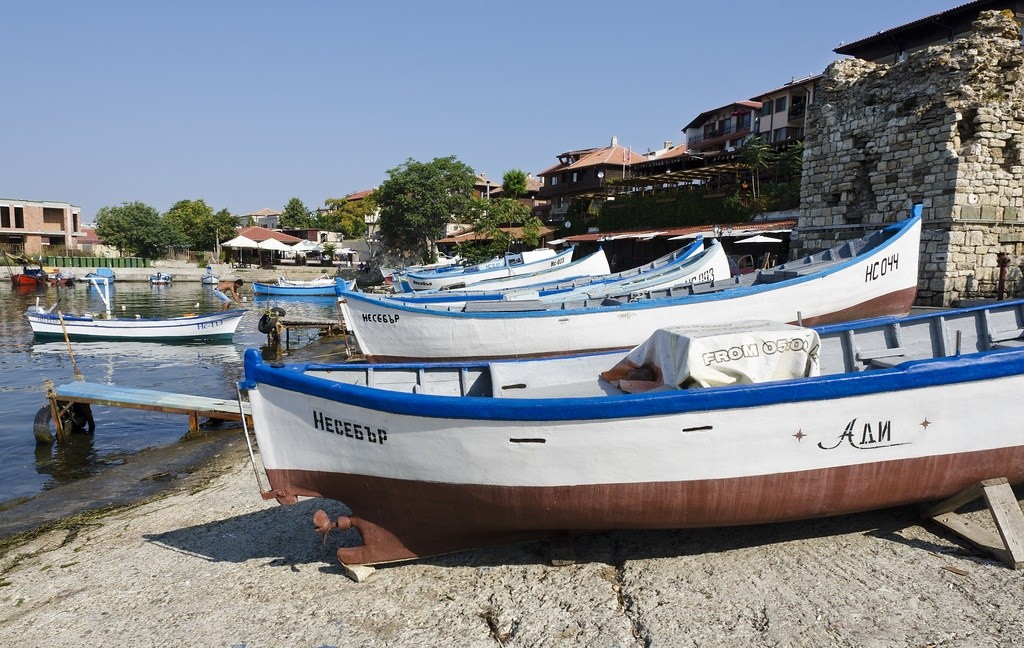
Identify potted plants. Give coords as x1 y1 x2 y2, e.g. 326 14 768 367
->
324 243 336 259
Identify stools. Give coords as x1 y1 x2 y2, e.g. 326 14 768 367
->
988 326 1024 351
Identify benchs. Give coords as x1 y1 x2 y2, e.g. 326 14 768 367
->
774 257 848 276
858 347 915 369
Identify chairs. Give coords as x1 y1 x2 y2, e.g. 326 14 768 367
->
231 260 252 268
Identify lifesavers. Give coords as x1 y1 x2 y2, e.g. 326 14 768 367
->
270 307 286 317
164 276 170 281
258 312 277 334
33 400 87 445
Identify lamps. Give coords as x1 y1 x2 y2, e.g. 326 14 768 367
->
666 168 671 173
564 221 572 229
597 172 604 178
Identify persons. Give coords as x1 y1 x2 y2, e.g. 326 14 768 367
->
213 279 244 312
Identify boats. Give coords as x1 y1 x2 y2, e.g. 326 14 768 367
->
85 267 116 284
199 264 219 286
148 272 173 285
232 298 1024 568
333 205 928 365
25 296 244 343
252 275 357 296
4 252 48 286
48 271 76 284
378 234 731 303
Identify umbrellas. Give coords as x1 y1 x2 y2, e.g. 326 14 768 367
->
220 235 258 263
257 238 289 262
734 235 783 254
292 239 323 265
